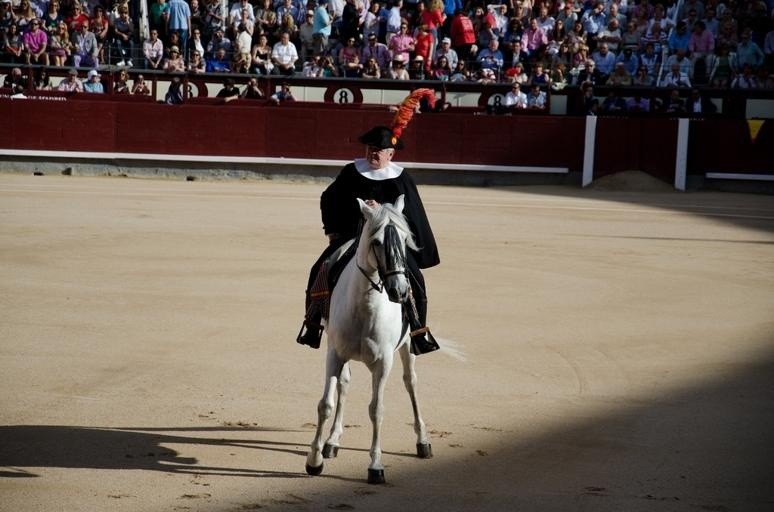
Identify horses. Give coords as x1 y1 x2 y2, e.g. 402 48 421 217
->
306 194 433 485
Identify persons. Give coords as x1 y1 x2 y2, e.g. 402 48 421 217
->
305 126 441 352
1 0 773 117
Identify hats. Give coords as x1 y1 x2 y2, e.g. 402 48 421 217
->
358 126 403 150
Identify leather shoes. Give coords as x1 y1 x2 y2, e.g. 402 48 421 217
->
299 329 320 348
413 334 436 355
